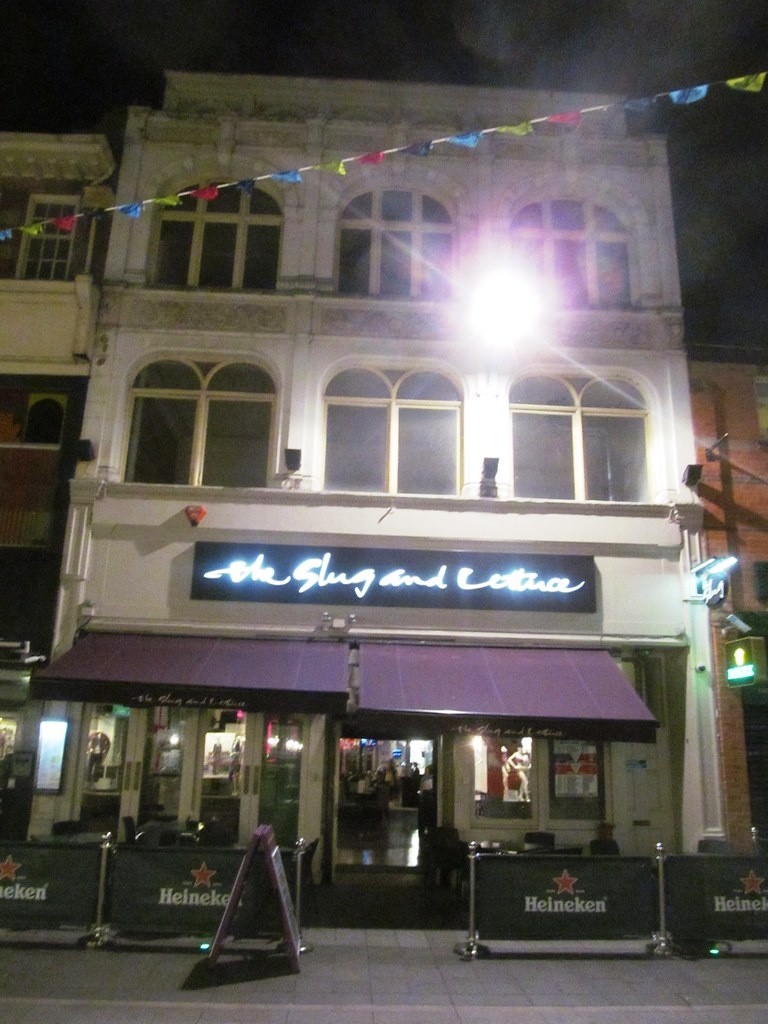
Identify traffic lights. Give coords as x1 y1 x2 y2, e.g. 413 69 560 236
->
724 636 767 690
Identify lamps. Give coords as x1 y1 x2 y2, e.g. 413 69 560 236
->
320 611 357 633
70 439 96 462
282 449 302 479
681 463 704 488
479 457 500 498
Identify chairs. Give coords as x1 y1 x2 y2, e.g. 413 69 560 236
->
589 838 619 854
697 839 733 852
122 815 138 844
523 832 557 850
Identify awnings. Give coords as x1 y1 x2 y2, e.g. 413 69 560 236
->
30 632 350 713
357 643 659 742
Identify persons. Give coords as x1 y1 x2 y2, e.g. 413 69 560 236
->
229 736 242 796
212 743 222 775
87 731 110 791
501 744 532 802
589 822 619 854
